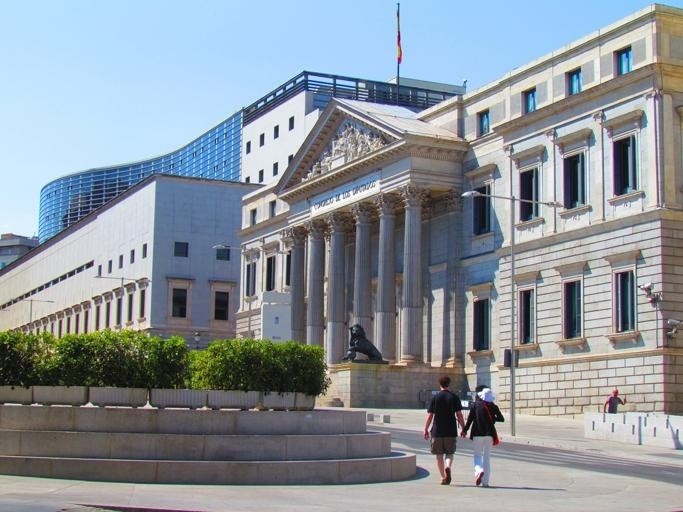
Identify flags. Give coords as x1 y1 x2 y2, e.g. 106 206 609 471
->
396 7 402 64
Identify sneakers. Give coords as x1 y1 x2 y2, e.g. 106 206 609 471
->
440 479 447 485
445 467 451 484
482 483 489 487
474 471 484 485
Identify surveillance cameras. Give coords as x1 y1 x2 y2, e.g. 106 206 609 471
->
666 319 680 327
640 282 654 290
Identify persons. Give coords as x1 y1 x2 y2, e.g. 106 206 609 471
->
460 387 504 488
424 376 466 485
604 388 627 414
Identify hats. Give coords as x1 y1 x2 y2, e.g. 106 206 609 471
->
477 388 495 402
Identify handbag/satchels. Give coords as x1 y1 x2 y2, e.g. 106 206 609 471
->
492 432 499 448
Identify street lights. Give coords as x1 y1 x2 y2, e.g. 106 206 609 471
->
461 190 563 436
10 298 55 331
93 276 141 332
212 244 291 338
